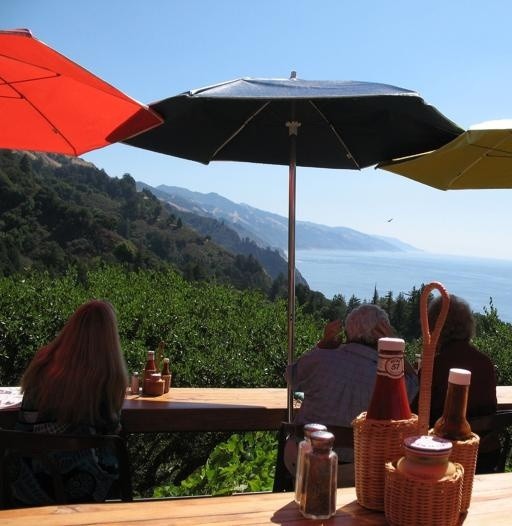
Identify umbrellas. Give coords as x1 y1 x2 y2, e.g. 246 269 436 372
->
104 67 469 429
0 25 166 157
367 116 512 193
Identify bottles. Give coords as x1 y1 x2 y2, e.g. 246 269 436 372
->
143 349 172 381
366 336 474 479
294 422 339 521
131 372 139 395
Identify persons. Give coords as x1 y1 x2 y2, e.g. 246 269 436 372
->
407 293 502 472
0 298 138 510
279 302 421 493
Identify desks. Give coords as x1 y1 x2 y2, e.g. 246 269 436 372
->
1 386 512 436
1 470 512 525
